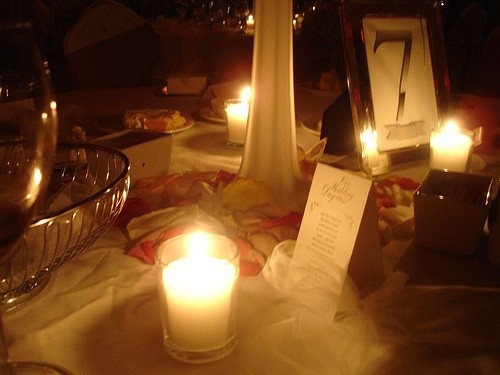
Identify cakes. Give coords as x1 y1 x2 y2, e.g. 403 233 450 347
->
122 108 186 131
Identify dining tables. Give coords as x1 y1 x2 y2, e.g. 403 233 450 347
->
1 82 500 374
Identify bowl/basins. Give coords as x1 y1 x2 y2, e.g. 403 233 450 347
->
0 142 131 313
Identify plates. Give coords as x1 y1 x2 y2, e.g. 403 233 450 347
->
124 109 195 133
200 104 226 124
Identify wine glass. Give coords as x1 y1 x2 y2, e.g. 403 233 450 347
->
0 53 74 375
